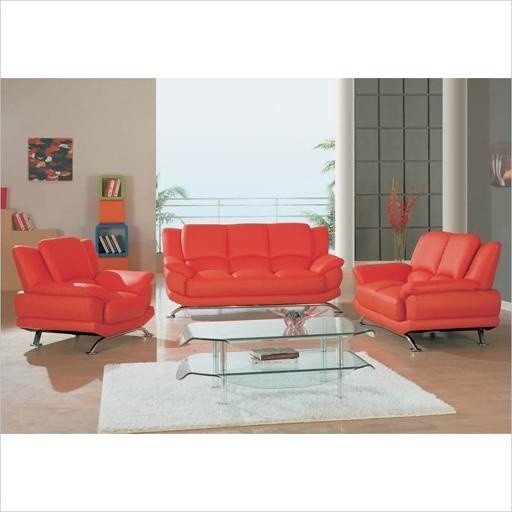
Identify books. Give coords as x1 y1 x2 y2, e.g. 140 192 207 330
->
98 233 123 254
249 346 300 361
12 212 32 231
104 178 121 197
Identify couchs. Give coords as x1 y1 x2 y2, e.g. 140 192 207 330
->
161 222 345 319
351 230 501 352
12 236 155 355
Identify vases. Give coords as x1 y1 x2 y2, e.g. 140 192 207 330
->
392 230 406 263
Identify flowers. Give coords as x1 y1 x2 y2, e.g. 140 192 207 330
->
385 176 426 247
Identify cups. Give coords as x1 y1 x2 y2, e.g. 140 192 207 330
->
0 186 8 209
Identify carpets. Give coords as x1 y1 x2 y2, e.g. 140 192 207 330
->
97 350 457 434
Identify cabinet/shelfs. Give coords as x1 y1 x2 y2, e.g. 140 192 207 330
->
95 174 128 270
0 209 61 291
